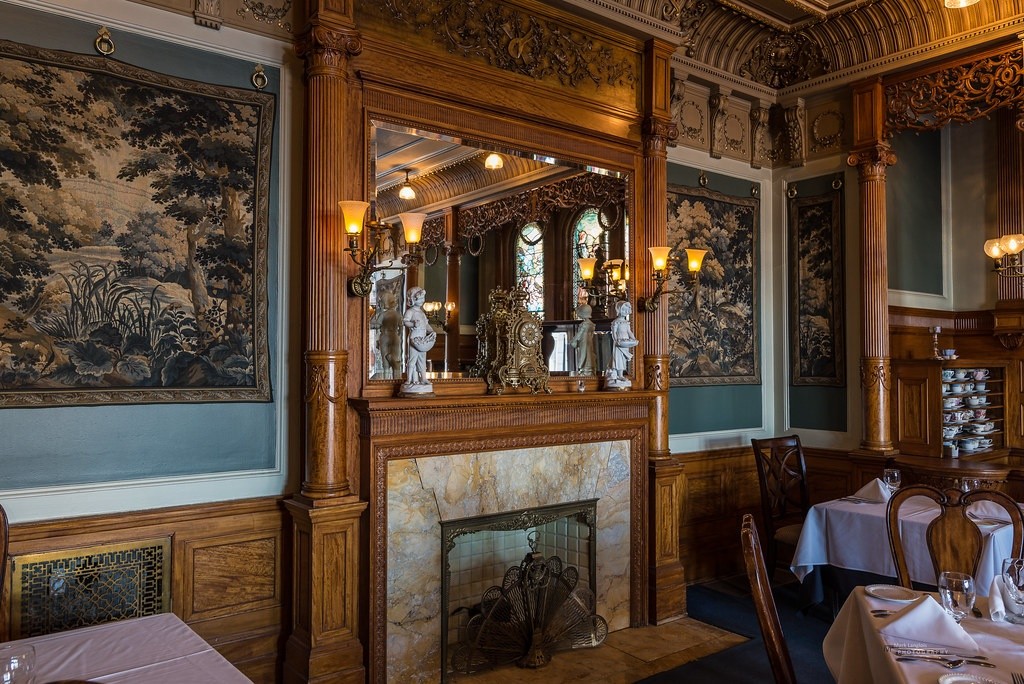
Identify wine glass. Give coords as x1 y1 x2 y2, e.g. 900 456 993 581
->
1002 558 1024 624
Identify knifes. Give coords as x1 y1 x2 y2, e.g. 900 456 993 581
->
894 652 996 668
884 645 989 660
967 593 982 618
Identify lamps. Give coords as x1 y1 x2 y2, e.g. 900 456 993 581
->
424 301 456 334
336 198 428 299
485 152 503 170
576 257 629 302
984 233 1024 278
398 169 417 200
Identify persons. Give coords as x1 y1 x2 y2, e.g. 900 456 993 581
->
372 289 402 381
612 301 639 389
396 285 439 396
572 304 599 377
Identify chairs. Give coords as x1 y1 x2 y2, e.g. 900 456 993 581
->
751 434 846 597
740 514 798 684
886 481 1024 593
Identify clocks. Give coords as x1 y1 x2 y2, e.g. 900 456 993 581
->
495 306 553 395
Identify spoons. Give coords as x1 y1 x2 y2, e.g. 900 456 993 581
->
896 657 965 669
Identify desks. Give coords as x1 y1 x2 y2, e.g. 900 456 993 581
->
823 583 1024 684
637 247 709 313
0 610 259 684
791 486 1024 596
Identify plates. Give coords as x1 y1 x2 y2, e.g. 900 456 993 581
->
942 377 992 382
937 673 999 684
864 584 920 602
942 390 994 452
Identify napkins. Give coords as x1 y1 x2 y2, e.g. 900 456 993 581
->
854 478 892 504
988 574 1024 623
879 594 980 652
972 498 1013 523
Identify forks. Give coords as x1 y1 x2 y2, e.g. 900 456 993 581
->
1011 672 1024 684
969 511 1013 525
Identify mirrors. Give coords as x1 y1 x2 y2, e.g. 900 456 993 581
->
361 102 638 385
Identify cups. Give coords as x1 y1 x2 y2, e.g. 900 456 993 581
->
962 477 980 492
884 468 901 495
938 571 976 625
942 368 989 380
942 383 994 449
0 644 37 684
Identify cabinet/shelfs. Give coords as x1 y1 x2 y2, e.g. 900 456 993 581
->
890 350 1024 459
540 317 615 377
